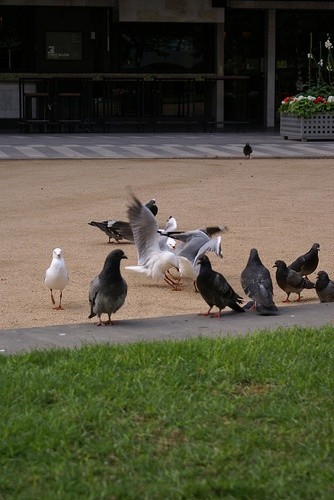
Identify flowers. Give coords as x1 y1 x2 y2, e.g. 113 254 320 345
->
278 31 334 117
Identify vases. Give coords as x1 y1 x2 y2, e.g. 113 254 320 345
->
279 110 334 142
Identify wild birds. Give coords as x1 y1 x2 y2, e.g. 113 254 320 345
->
196 254 246 319
87 198 229 292
44 248 68 310
272 259 318 303
288 242 321 282
241 248 279 314
243 142 253 160
315 270 334 303
88 248 128 327
